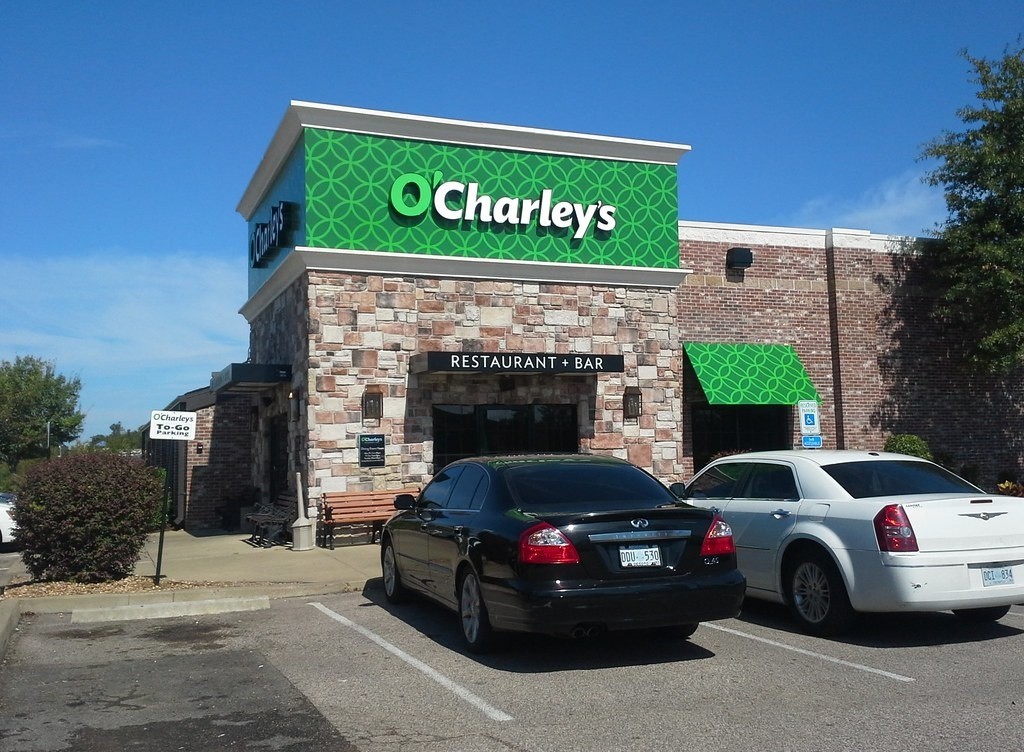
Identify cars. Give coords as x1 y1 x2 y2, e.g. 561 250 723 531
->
379 453 747 651
0 492 19 544
669 448 1024 629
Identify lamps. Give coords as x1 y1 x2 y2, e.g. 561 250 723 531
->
727 247 753 269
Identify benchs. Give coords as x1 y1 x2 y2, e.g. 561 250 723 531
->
320 486 422 550
244 495 297 549
